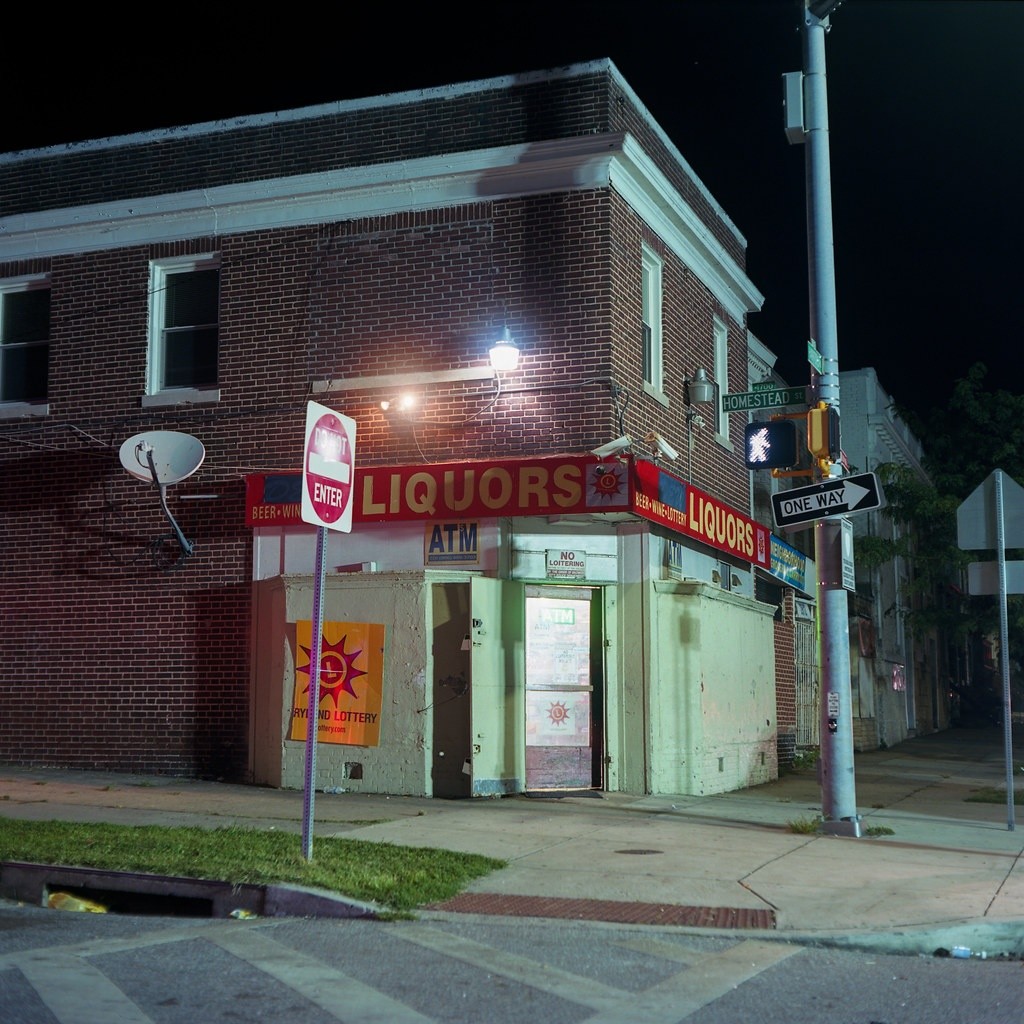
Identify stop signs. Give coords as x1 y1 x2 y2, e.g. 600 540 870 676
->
300 400 357 534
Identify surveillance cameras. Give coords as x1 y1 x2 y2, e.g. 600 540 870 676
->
647 432 679 461
591 436 632 459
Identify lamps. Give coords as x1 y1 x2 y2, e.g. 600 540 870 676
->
686 365 715 404
487 320 520 371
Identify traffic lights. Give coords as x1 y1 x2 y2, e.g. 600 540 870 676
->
744 419 796 470
807 406 844 461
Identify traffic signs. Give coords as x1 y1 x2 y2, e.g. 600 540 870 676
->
771 472 881 528
